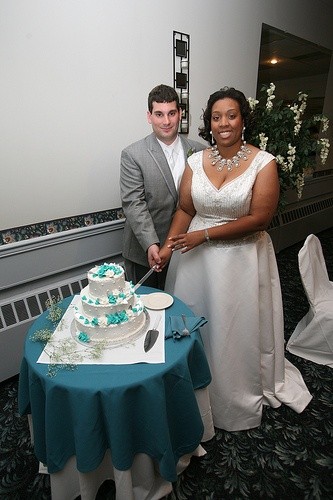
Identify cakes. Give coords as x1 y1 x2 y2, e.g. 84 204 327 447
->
73 263 144 342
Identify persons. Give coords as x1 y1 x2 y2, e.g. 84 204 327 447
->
148 87 313 440
121 84 212 293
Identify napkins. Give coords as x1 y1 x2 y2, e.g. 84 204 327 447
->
165 315 209 343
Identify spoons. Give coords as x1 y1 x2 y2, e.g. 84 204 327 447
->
181 314 190 337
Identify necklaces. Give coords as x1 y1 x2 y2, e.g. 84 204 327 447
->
206 138 252 172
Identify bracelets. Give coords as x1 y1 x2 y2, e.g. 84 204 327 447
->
204 228 209 242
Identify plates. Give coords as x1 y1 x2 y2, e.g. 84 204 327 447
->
143 293 175 310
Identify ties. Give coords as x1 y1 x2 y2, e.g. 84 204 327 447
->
167 148 181 194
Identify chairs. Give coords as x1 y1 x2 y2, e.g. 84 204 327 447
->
286 234 333 370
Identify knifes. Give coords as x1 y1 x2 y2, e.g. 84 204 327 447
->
134 262 158 292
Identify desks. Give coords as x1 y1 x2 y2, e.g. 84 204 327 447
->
18 284 216 500
